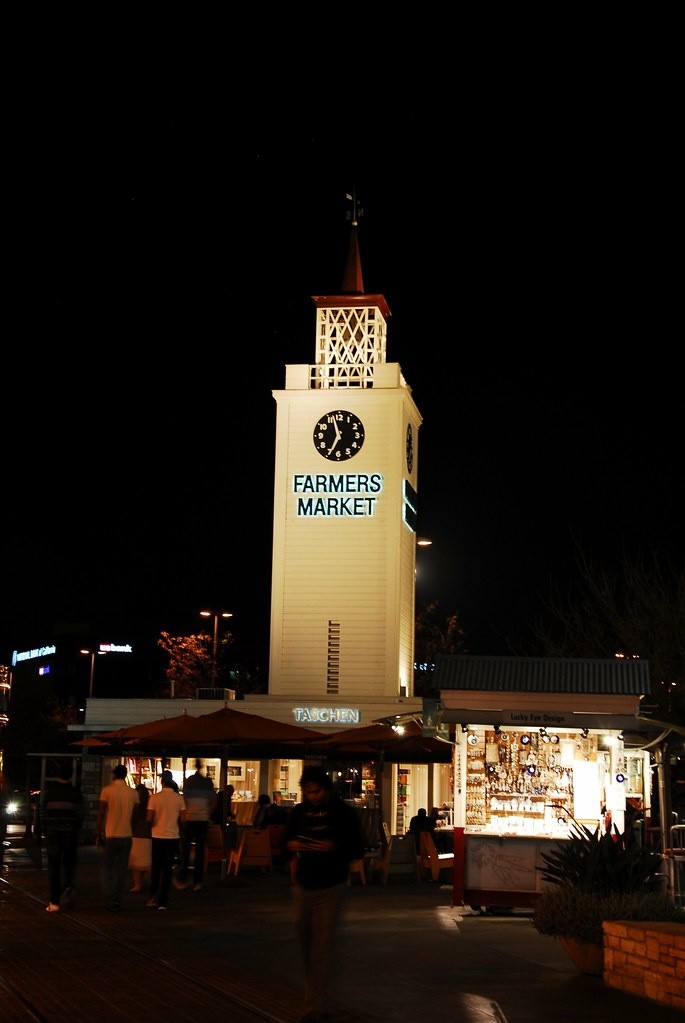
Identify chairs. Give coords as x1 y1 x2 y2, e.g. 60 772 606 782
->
202 823 454 888
660 824 685 909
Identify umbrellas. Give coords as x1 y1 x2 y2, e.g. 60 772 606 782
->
70 704 453 807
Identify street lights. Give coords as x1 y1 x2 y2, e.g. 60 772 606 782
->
80 649 106 699
199 609 233 689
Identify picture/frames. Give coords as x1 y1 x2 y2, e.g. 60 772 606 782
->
202 762 220 789
228 761 246 781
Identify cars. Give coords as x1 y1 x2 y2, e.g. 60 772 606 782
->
4 783 38 825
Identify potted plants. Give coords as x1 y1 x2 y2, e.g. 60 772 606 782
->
529 885 685 978
158 631 213 700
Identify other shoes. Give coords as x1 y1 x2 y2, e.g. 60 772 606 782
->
158 902 166 909
46 904 59 911
64 888 72 897
146 899 156 906
106 902 120 910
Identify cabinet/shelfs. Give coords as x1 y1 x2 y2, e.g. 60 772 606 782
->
106 757 157 795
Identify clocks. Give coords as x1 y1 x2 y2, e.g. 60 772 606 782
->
405 422 413 474
313 410 365 462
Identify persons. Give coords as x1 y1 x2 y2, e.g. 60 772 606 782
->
409 808 444 889
175 759 216 891
253 794 276 829
36 767 85 913
209 786 237 833
143 771 188 912
94 765 136 912
276 766 367 1023
129 783 156 894
